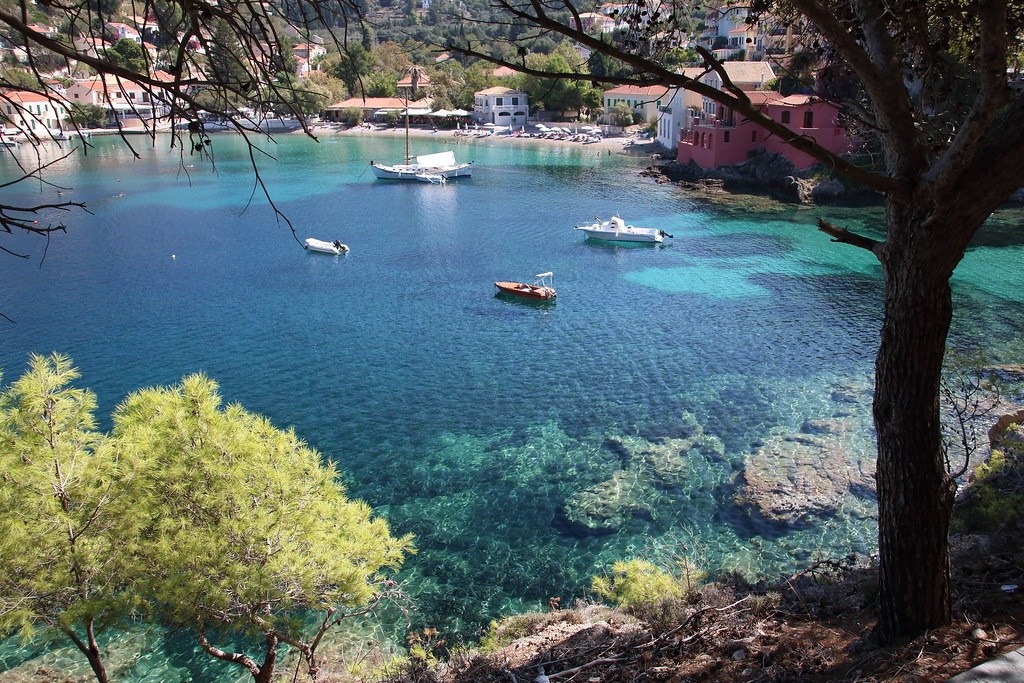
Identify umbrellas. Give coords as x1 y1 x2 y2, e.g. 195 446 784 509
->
533 124 603 135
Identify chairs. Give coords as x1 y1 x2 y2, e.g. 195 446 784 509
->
500 131 596 144
452 130 493 138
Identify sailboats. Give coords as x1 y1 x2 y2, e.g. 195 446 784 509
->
369 86 474 179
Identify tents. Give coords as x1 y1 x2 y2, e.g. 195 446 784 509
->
374 109 474 117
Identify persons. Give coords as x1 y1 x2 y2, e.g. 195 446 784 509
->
364 114 474 127
335 239 342 249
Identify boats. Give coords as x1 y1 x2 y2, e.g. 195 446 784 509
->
412 172 447 185
572 211 675 243
303 238 350 254
494 272 558 300
